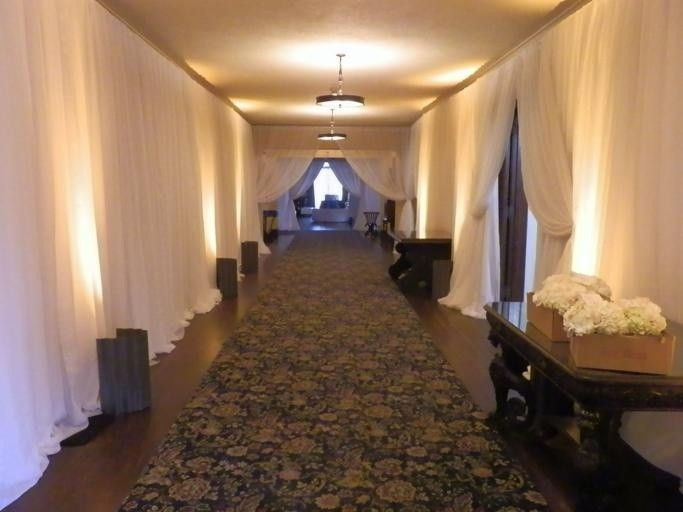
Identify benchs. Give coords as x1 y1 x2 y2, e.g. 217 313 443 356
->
484 299 683 500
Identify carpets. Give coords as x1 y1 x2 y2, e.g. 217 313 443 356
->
117 233 549 512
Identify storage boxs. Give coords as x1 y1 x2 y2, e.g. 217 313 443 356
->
525 291 568 344
570 331 675 377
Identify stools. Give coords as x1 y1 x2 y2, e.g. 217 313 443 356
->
239 240 258 273
215 256 237 300
96 328 150 417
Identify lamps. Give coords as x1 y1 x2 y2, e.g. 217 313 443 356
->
318 112 347 141
315 53 366 112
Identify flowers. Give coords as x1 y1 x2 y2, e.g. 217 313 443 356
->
532 269 611 312
560 294 665 336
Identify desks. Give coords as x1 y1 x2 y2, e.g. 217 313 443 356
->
360 211 379 237
385 238 453 302
